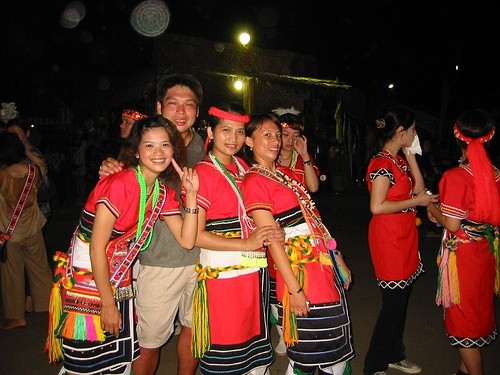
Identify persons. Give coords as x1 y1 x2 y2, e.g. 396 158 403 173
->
427 108 500 375
180 102 286 375
39 117 200 375
271 106 321 193
362 106 438 375
0 95 155 330
99 73 208 375
242 108 355 375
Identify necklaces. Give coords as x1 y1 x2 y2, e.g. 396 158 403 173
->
137 164 159 251
208 151 257 240
278 148 298 170
251 164 316 210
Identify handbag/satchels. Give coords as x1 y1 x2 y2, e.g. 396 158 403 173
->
53 283 116 342
0 241 7 263
334 254 352 290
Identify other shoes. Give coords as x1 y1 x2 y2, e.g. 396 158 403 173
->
274 336 287 356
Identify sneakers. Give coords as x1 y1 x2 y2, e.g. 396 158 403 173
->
388 359 421 373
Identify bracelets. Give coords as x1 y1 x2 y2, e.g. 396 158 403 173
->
182 203 199 214
301 160 311 165
288 287 303 295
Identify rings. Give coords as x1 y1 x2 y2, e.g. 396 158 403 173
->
262 240 266 248
298 310 303 314
99 166 103 170
